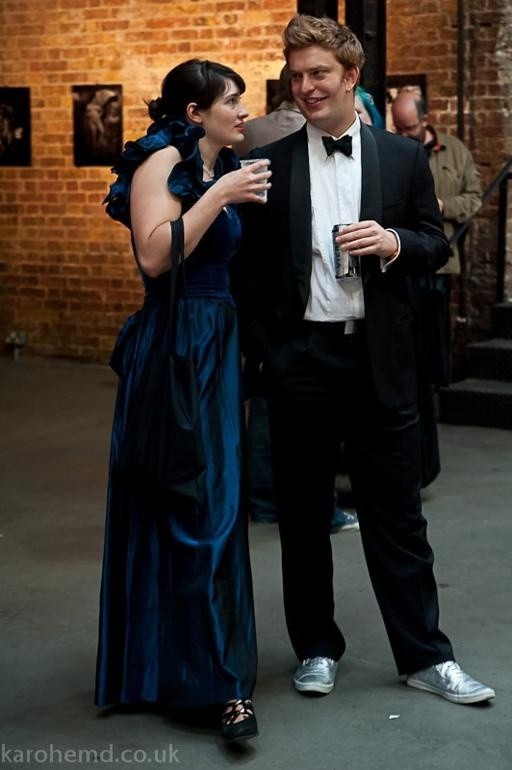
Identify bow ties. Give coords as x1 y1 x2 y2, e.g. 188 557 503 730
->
321 134 353 159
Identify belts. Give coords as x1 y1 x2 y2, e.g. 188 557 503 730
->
298 316 366 340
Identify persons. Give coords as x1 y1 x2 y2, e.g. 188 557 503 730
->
237 10 500 706
91 55 276 749
386 86 483 378
200 67 362 536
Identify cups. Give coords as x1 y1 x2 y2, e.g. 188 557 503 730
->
240 159 270 205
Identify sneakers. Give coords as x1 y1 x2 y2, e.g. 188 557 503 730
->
291 652 339 698
219 691 259 744
404 656 497 705
334 509 362 529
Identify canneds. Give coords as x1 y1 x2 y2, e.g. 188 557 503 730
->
331 223 360 283
